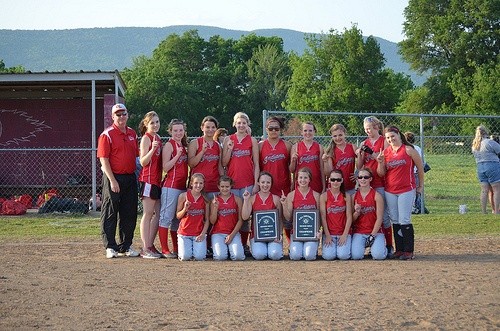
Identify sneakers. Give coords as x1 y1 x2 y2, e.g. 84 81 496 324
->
393 252 402 258
118 249 140 257
399 253 415 260
143 250 161 259
140 249 162 256
107 249 118 259
387 246 394 257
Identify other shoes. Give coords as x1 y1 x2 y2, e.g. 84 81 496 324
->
171 251 178 255
420 209 429 214
161 252 178 258
411 209 418 214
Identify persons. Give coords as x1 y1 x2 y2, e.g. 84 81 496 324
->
471 125 500 214
321 123 360 198
140 111 162 260
209 175 246 261
242 171 283 260
355 116 394 259
176 172 209 260
98 103 140 258
258 117 294 257
405 129 430 215
378 125 424 260
213 125 251 176
280 167 321 261
352 168 387 260
489 133 500 143
320 169 352 260
222 112 260 256
159 119 189 259
488 186 495 214
188 115 223 256
289 121 326 193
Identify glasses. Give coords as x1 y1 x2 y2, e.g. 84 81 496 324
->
364 118 378 125
329 178 343 183
358 175 370 179
268 125 281 132
115 112 128 117
303 129 315 132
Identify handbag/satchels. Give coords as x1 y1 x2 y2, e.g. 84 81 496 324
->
423 163 431 173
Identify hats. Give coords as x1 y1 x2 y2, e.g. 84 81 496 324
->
112 104 127 113
